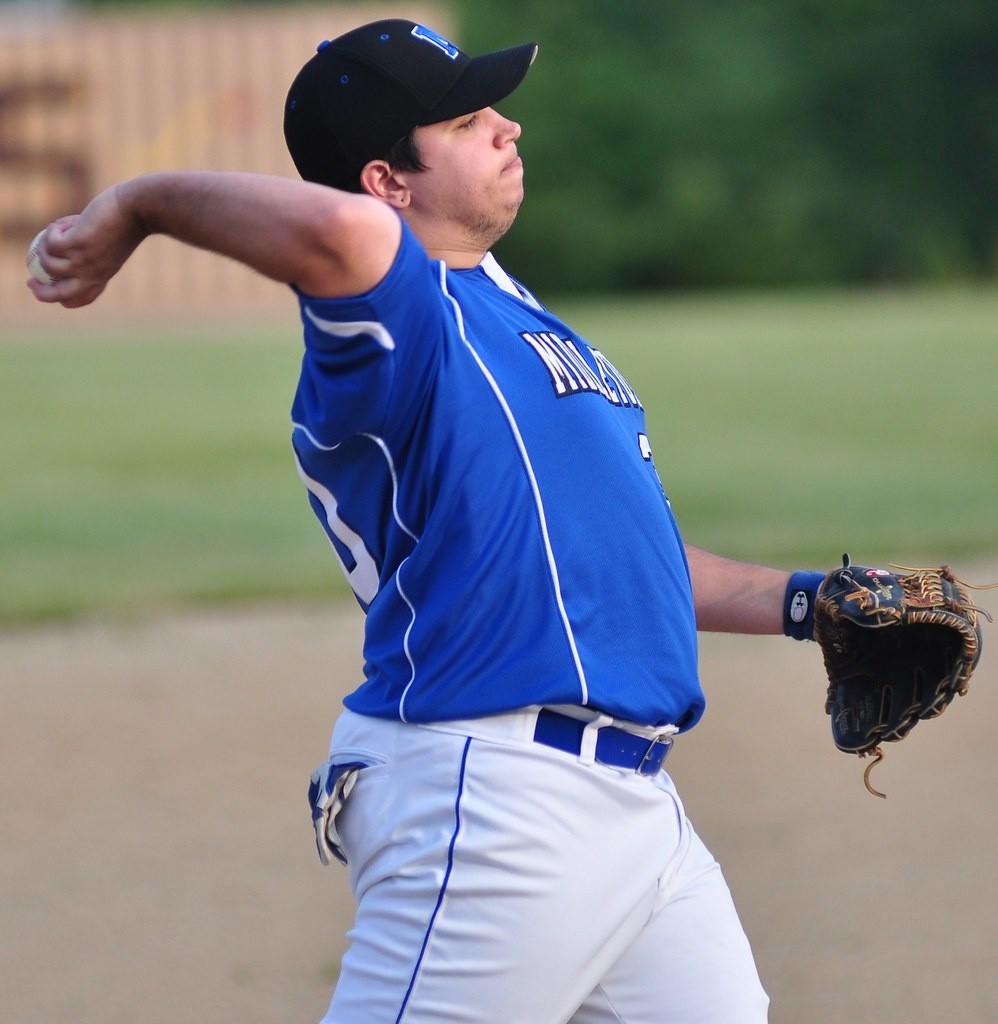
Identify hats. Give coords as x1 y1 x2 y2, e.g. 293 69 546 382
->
284 18 539 192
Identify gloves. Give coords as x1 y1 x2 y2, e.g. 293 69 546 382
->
308 761 359 868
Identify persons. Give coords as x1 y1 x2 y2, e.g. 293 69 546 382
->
26 18 984 1024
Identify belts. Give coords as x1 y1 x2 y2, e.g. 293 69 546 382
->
534 709 673 775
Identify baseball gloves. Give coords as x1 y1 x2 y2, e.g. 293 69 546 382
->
778 548 998 800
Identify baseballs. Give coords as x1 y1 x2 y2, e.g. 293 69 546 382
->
24 227 63 286
